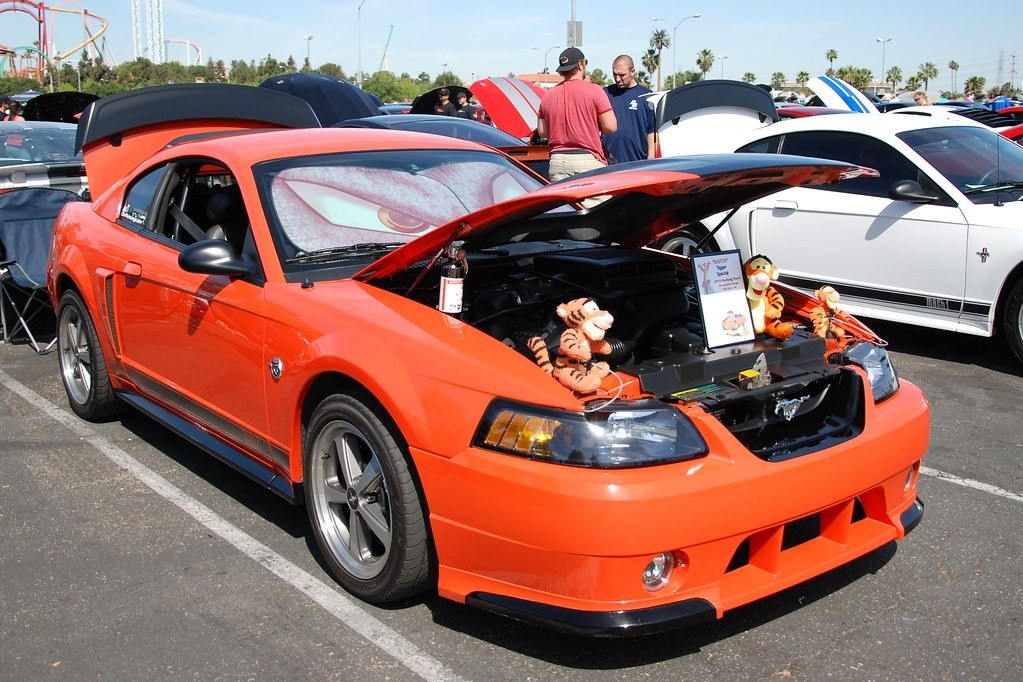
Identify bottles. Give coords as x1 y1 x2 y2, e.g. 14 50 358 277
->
438 244 466 320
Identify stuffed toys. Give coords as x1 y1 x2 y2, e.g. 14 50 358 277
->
527 296 614 394
743 253 793 340
810 287 850 341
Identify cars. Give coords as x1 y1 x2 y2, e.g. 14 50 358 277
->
8 90 102 124
650 80 1023 382
766 76 1022 182
0 121 92 332
257 72 532 149
46 81 930 641
365 75 552 143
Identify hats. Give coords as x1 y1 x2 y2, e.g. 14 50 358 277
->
457 92 466 98
556 48 584 72
438 88 450 95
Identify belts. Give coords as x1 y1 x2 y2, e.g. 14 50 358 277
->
553 149 590 153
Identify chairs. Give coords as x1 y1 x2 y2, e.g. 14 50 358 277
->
0 187 87 358
168 183 243 257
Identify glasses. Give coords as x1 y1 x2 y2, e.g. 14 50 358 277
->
580 59 588 65
439 93 448 96
458 98 465 100
915 98 919 102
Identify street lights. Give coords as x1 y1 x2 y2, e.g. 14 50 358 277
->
652 14 700 87
719 54 729 80
877 36 895 80
528 44 561 74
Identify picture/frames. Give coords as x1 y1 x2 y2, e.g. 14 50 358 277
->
689 249 758 350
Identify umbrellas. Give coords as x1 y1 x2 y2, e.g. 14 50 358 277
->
933 96 975 102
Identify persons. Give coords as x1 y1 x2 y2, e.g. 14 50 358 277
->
537 47 618 208
989 93 995 99
3 101 25 149
0 95 11 145
433 88 457 137
601 55 658 166
456 92 476 138
992 91 1011 111
788 93 798 103
913 91 932 106
1010 96 1021 107
